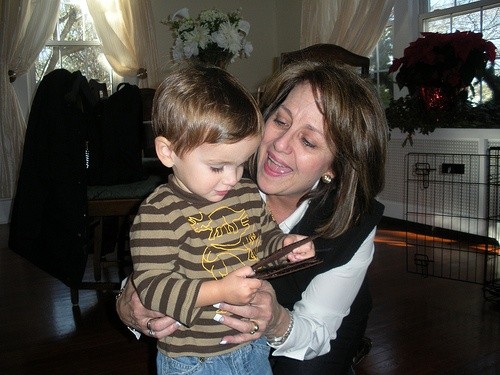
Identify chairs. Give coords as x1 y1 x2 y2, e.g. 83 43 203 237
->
8 68 167 304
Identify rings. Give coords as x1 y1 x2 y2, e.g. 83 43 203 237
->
149 330 155 338
146 319 154 331
250 320 259 335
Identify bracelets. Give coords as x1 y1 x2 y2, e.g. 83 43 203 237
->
265 305 285 336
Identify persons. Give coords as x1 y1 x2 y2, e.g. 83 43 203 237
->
129 65 315 375
114 61 390 375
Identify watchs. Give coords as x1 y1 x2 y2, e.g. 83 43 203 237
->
267 308 294 346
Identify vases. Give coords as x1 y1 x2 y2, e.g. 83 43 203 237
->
420 84 459 112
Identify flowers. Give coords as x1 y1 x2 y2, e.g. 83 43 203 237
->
161 6 253 68
388 30 497 92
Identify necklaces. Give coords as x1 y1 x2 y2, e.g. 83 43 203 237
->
267 203 277 223
